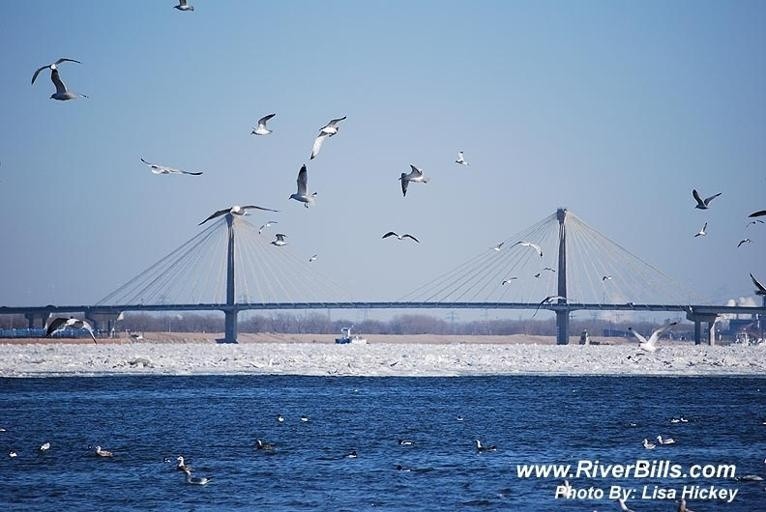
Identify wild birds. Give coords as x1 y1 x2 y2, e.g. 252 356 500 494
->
0 413 766 512
31 58 91 100
693 189 722 209
745 220 765 229
750 273 766 296
173 0 194 11
46 318 97 346
738 239 751 247
695 222 707 238
492 240 676 354
140 113 470 263
